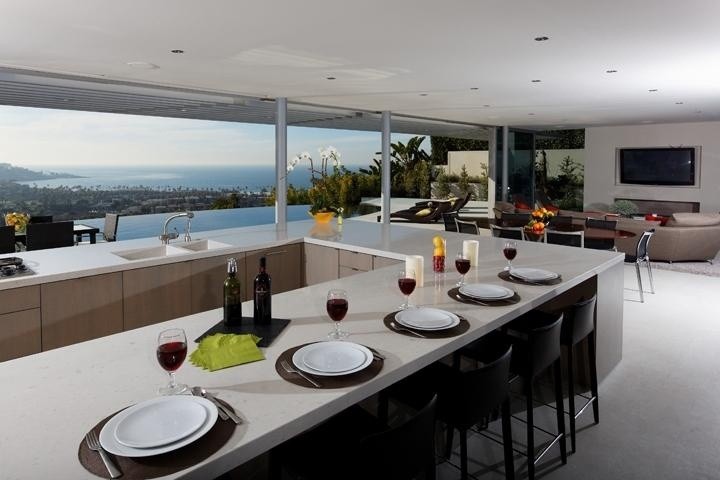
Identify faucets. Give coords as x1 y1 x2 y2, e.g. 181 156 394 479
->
158 212 195 244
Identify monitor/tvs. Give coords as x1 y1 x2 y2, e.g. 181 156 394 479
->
618 147 696 188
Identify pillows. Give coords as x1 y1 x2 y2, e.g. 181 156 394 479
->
449 199 455 206
667 212 717 229
646 215 669 226
415 207 433 217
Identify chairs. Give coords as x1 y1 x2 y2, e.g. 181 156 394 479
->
542 230 585 252
78 214 119 245
446 309 571 480
442 211 466 237
547 216 573 227
454 222 480 235
28 214 52 225
486 225 524 244
0 226 15 254
500 295 600 457
585 220 618 252
623 230 654 298
27 221 74 250
266 401 436 480
376 344 523 480
500 213 532 226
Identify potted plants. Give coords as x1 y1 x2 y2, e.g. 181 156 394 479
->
615 200 640 219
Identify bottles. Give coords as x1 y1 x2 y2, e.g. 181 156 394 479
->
223 257 243 328
253 256 272 328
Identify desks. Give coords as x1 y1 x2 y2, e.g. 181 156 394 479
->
489 217 635 240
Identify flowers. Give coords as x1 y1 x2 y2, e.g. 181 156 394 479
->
279 149 337 214
337 207 344 217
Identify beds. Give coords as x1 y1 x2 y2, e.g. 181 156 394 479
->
415 191 473 212
377 202 451 223
410 198 466 221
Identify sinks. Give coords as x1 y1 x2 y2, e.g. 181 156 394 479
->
110 244 190 261
171 238 234 253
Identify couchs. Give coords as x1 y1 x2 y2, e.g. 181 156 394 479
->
492 201 719 263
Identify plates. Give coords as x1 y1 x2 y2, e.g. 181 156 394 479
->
403 311 452 329
98 393 219 457
396 309 459 331
115 400 207 447
459 284 514 299
293 341 373 376
468 286 508 298
302 346 366 371
512 268 555 280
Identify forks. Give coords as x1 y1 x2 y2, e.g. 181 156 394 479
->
508 274 542 287
280 359 318 387
85 431 124 478
455 293 489 306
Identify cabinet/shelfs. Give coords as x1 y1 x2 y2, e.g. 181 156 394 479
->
0 284 42 359
123 264 192 330
245 243 304 300
41 271 123 351
304 244 341 286
339 250 373 277
191 254 247 313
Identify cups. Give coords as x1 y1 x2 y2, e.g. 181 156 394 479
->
503 241 516 271
156 329 187 395
454 253 470 290
465 239 478 268
397 271 416 311
405 255 424 289
327 290 350 341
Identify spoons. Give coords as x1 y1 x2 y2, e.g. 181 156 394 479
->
193 386 242 425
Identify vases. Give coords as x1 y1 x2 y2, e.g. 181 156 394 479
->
307 210 337 226
337 217 342 224
